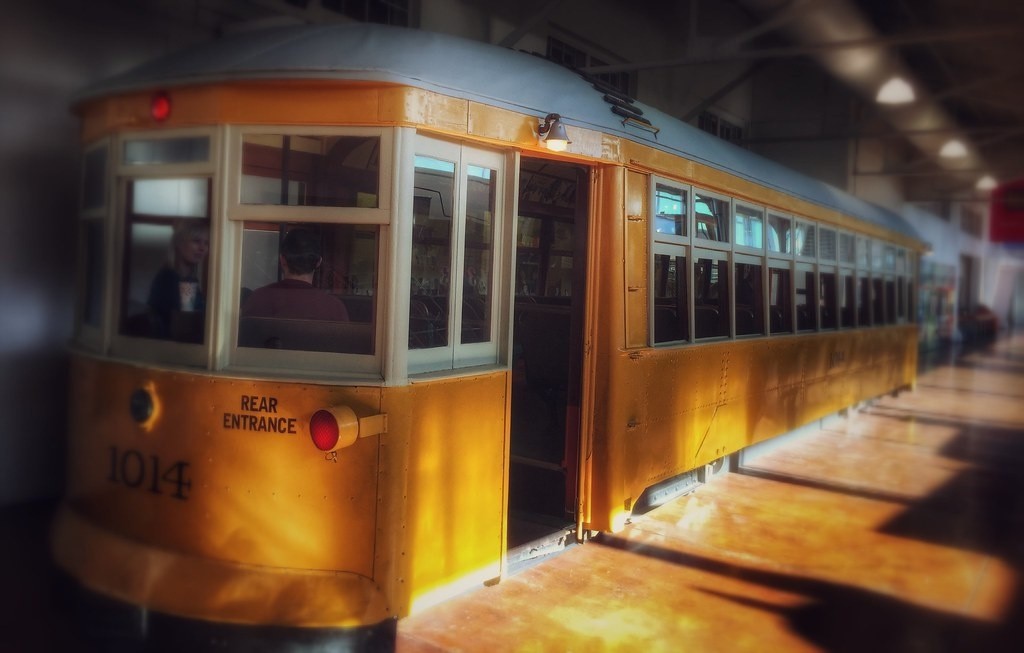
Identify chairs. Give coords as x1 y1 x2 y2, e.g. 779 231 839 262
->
412 294 570 464
655 305 830 342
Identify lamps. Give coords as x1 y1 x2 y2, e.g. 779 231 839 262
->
538 114 573 152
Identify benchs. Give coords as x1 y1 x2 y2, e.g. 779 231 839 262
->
171 309 375 355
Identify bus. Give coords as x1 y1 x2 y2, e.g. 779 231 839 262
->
47 9 934 629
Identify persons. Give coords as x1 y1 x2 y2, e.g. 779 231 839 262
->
145 224 209 313
237 228 350 323
